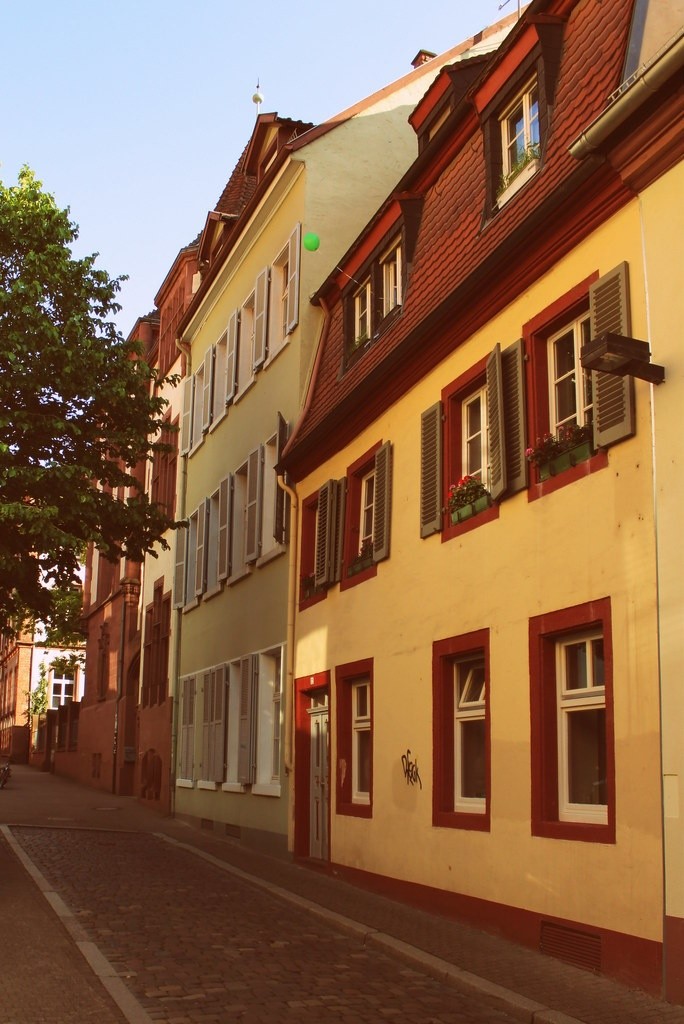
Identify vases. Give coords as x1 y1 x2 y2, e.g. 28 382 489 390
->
538 439 593 480
346 557 373 575
449 495 491 524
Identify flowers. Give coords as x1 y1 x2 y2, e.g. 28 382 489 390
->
526 424 592 464
352 542 372 562
446 476 487 510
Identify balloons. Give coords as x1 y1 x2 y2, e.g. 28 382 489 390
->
303 232 320 251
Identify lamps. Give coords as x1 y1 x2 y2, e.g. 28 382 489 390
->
581 333 666 386
